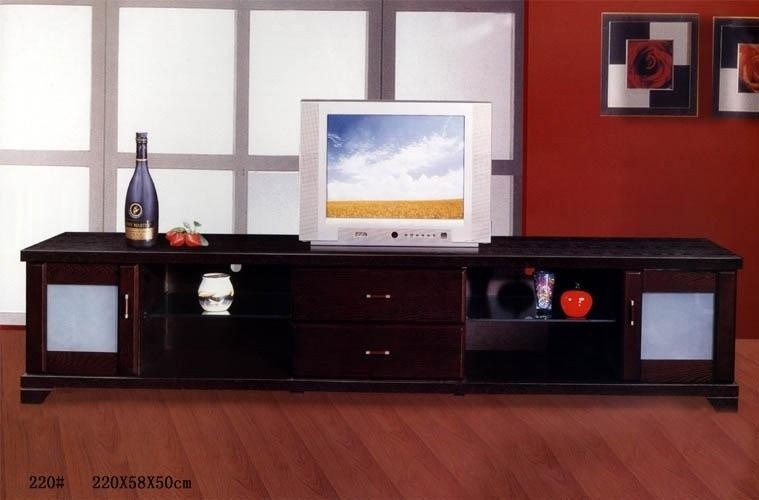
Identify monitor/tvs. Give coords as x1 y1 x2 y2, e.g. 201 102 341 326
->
299 100 493 255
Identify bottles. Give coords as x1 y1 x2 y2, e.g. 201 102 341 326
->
124 131 159 250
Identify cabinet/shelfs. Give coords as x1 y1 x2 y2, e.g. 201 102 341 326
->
12 231 748 418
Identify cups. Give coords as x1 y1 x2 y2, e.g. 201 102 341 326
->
531 269 557 320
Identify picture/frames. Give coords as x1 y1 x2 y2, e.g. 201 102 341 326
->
598 12 759 122
